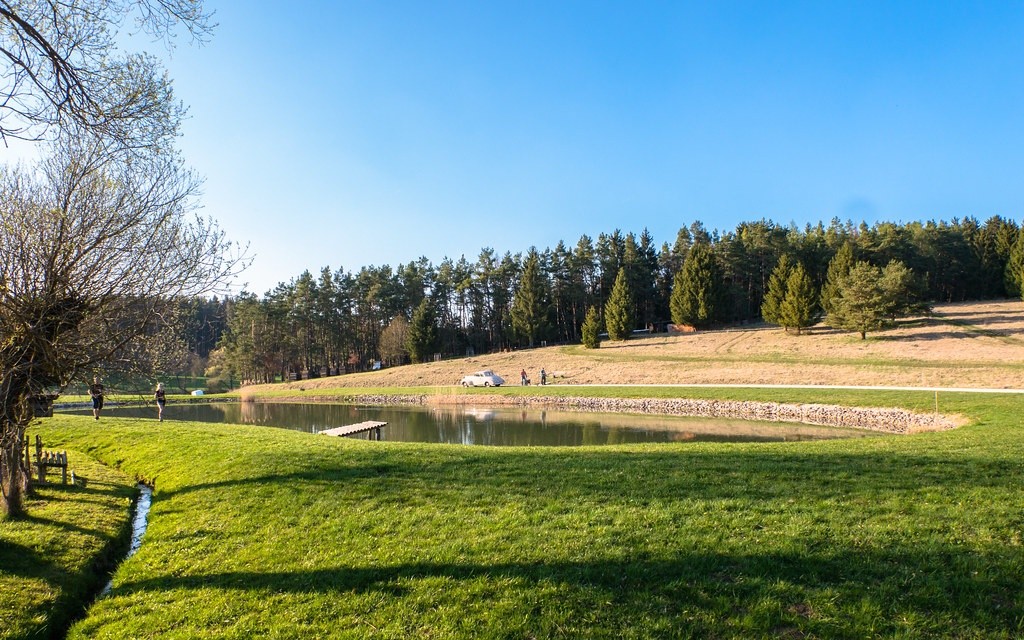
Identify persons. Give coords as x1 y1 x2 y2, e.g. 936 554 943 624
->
154 382 166 421
88 377 105 420
541 368 546 385
521 369 527 386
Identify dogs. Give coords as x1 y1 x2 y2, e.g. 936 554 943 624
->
525 379 531 385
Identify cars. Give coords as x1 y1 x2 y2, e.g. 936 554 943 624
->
463 409 495 421
461 371 504 387
